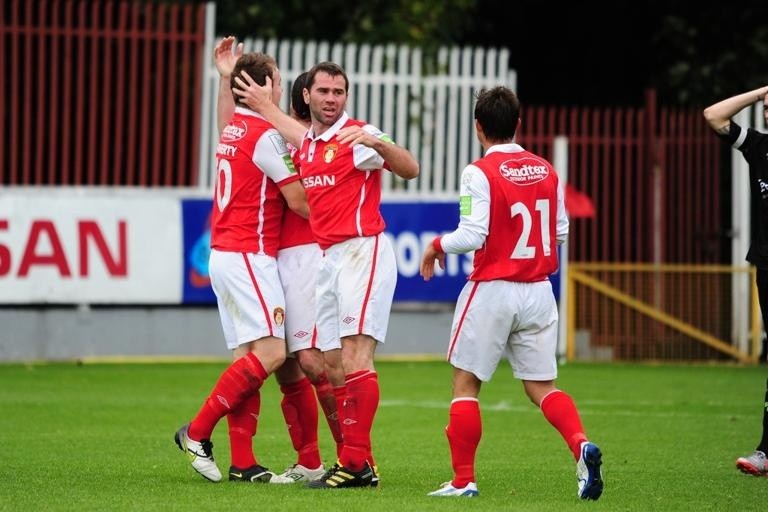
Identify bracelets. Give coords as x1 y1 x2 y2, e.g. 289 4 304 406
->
432 236 444 255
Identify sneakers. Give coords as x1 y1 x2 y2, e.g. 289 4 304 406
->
735 451 768 476
427 480 478 497
175 422 379 488
576 442 603 500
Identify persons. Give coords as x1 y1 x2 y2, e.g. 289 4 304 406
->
700 78 767 478
294 64 422 487
418 87 605 503
175 56 312 484
210 32 344 481
